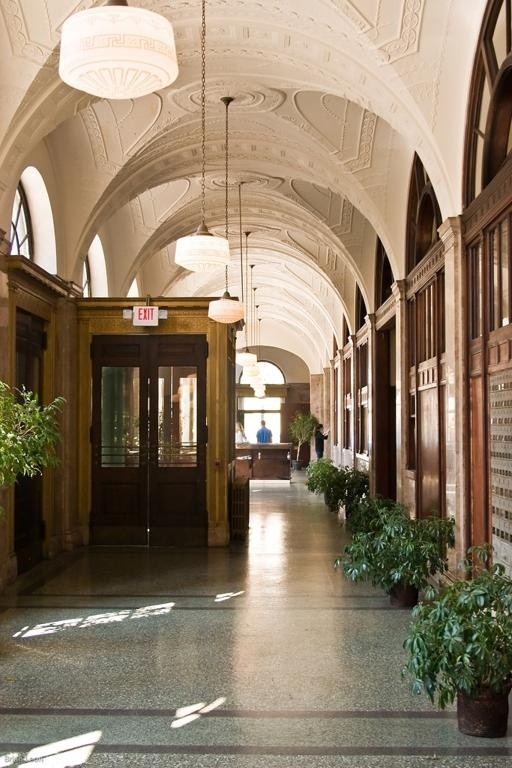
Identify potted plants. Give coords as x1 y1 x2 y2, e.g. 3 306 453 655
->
289 413 320 471
400 542 511 738
304 457 391 535
334 499 456 610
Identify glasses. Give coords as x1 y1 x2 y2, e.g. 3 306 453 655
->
174 0 271 399
57 0 179 103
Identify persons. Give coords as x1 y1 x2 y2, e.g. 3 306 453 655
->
257 420 272 444
314 424 329 461
235 421 247 444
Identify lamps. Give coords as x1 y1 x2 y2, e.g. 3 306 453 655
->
174 0 271 399
57 0 179 103
